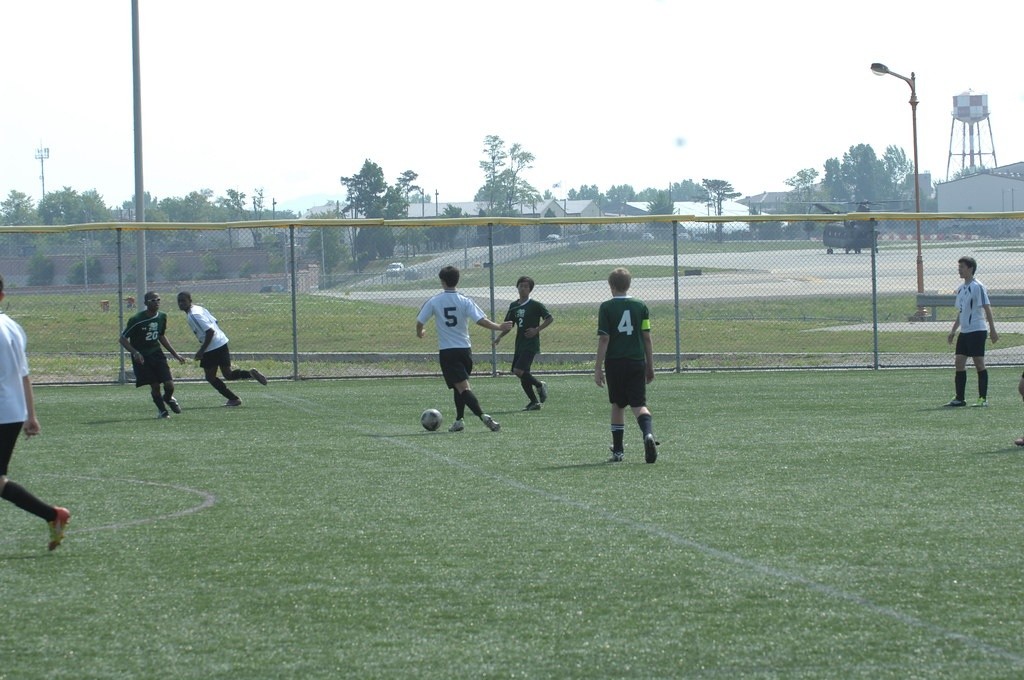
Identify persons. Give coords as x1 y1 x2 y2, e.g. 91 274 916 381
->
177 291 268 406
1014 370 1024 446
118 291 186 418
594 267 657 463
942 257 998 407
0 274 71 552
492 276 553 411
414 266 513 432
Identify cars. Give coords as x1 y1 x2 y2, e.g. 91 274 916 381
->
546 234 562 244
386 262 404 277
679 233 691 242
641 233 655 240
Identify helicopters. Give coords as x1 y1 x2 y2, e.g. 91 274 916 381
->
786 199 921 255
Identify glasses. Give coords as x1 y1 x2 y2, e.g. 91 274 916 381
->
148 299 160 302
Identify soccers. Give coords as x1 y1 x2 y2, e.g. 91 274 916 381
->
420 408 443 431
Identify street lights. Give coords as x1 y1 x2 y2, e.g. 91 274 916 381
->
200 235 208 251
872 63 925 320
278 232 288 290
560 199 566 241
705 192 710 236
435 193 440 217
82 238 88 289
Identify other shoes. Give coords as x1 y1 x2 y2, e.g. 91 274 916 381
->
1015 438 1024 446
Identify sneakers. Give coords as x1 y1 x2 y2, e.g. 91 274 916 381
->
609 448 624 461
971 397 988 407
943 397 967 406
523 402 540 410
156 410 173 420
163 394 181 413
481 413 500 431
645 433 660 463
537 381 547 403
49 508 70 551
449 417 465 431
224 396 241 406
250 368 267 385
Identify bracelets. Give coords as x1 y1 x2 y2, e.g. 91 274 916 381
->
133 351 140 358
